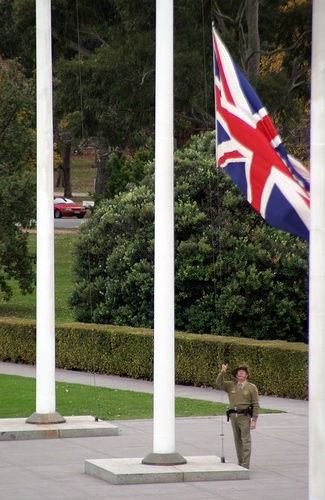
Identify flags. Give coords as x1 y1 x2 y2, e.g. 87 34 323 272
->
213 25 311 240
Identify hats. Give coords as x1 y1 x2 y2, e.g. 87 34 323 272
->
230 364 251 380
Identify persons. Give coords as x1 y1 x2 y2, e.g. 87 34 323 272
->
216 364 259 469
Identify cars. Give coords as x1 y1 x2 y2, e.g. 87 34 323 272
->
54 195 87 220
